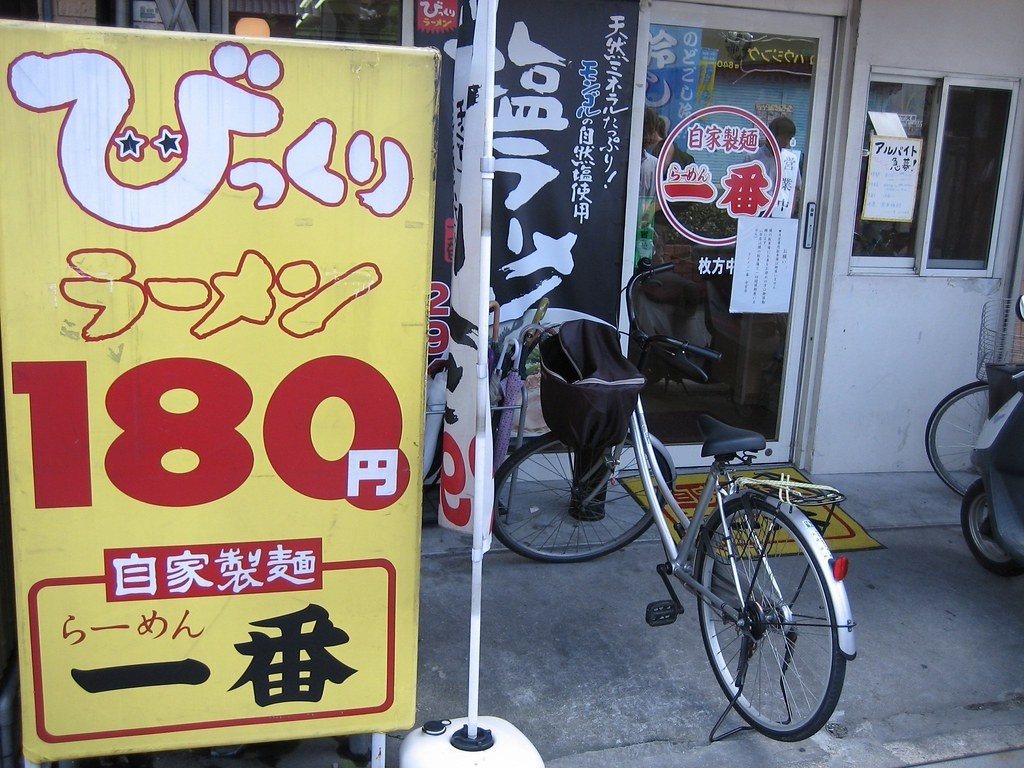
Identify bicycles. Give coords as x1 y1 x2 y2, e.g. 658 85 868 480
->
492 257 856 742
926 298 1024 497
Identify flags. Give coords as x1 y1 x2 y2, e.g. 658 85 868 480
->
434 1 502 536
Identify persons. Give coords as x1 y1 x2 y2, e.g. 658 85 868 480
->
640 111 801 255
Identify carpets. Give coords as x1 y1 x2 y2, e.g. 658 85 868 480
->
617 464 888 563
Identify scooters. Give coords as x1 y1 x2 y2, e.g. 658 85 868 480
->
961 294 1024 577
630 210 787 423
